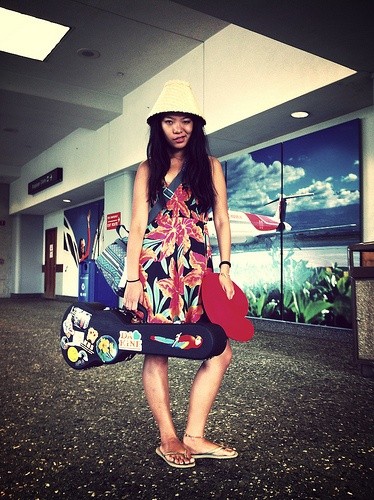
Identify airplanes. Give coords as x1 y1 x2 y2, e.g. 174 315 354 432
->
202 193 314 252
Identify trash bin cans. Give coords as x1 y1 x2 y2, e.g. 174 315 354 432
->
346 240 374 378
78 259 118 307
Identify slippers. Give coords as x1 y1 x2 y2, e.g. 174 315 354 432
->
182 443 239 458
156 447 196 468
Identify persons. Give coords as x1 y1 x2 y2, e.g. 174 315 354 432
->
123 79 239 469
77 208 92 261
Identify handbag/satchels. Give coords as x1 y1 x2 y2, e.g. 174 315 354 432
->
95 224 130 299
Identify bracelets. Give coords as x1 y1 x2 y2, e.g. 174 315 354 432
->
126 278 140 282
219 260 231 269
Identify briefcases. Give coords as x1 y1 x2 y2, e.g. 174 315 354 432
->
61 302 228 370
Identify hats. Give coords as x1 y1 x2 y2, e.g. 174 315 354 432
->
147 79 207 126
203 268 255 342
